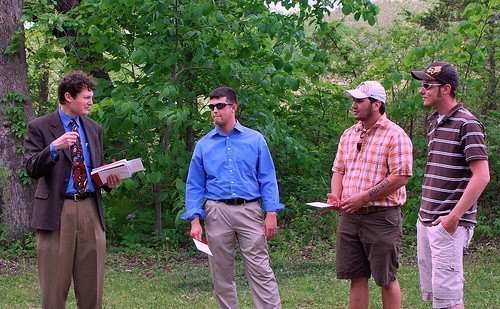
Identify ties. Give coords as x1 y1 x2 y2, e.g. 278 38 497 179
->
69 119 87 196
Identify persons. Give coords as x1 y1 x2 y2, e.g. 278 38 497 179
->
25 70 121 309
326 80 413 309
411 61 490 309
182 87 285 309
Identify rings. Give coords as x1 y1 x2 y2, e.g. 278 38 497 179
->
348 209 350 212
111 183 114 184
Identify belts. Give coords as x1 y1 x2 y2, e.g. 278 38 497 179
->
64 194 95 202
356 206 400 214
213 198 259 205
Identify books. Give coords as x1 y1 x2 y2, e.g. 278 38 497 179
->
91 159 132 186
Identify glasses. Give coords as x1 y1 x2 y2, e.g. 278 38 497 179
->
357 131 367 151
207 103 233 110
421 83 442 90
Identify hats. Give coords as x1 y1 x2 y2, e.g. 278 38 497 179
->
411 61 459 91
342 80 387 104
